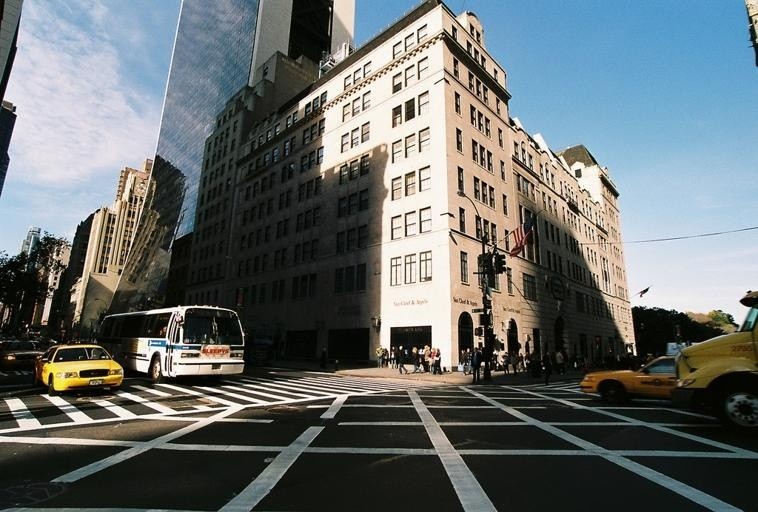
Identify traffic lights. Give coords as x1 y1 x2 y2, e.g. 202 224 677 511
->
496 253 506 274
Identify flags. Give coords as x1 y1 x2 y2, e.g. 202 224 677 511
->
506 213 535 259
640 288 649 298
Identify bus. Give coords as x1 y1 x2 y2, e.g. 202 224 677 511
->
98 305 246 383
675 291 758 437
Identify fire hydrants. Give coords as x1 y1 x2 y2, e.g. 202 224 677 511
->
335 360 339 371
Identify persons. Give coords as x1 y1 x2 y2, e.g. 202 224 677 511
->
371 334 637 387
320 345 329 368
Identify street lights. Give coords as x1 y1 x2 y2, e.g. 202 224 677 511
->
95 298 108 313
457 191 492 381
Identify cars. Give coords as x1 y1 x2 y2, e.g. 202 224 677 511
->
580 355 675 403
0 342 125 396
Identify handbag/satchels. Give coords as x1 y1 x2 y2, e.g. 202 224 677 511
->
502 364 507 369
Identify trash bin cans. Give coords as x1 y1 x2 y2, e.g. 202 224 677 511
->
530 359 543 378
457 364 464 372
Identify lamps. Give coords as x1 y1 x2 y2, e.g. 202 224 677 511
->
371 315 380 332
315 319 322 335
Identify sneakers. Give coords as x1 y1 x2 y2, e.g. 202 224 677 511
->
399 370 442 376
